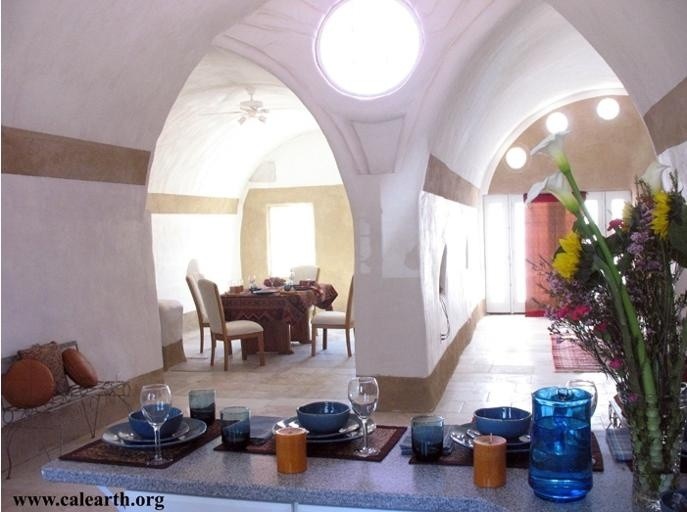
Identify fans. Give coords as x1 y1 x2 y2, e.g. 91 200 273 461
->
199 89 301 124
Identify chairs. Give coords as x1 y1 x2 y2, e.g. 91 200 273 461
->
311 275 354 359
197 279 267 373
290 266 318 337
186 268 211 354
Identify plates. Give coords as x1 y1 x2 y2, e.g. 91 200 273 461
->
467 426 532 446
254 291 273 294
275 419 359 438
102 416 208 448
117 423 189 443
272 416 376 443
450 421 532 451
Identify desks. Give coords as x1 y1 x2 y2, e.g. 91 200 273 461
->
222 283 338 354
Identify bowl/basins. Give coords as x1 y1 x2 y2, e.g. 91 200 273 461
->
130 407 183 437
473 407 531 439
296 400 351 432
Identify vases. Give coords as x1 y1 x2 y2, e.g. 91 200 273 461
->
623 389 687 512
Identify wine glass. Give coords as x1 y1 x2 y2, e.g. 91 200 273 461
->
566 381 597 465
289 272 296 284
141 384 172 466
248 273 255 295
348 377 380 458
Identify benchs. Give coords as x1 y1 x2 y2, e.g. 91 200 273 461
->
1 340 132 480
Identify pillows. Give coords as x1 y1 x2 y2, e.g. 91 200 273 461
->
19 341 69 395
3 361 55 408
63 349 98 387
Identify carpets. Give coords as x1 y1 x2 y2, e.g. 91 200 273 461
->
550 330 607 373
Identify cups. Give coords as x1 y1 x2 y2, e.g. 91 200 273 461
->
220 406 250 450
189 389 215 425
528 385 593 504
410 415 445 460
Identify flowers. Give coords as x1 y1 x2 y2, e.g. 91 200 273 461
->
522 129 687 512
